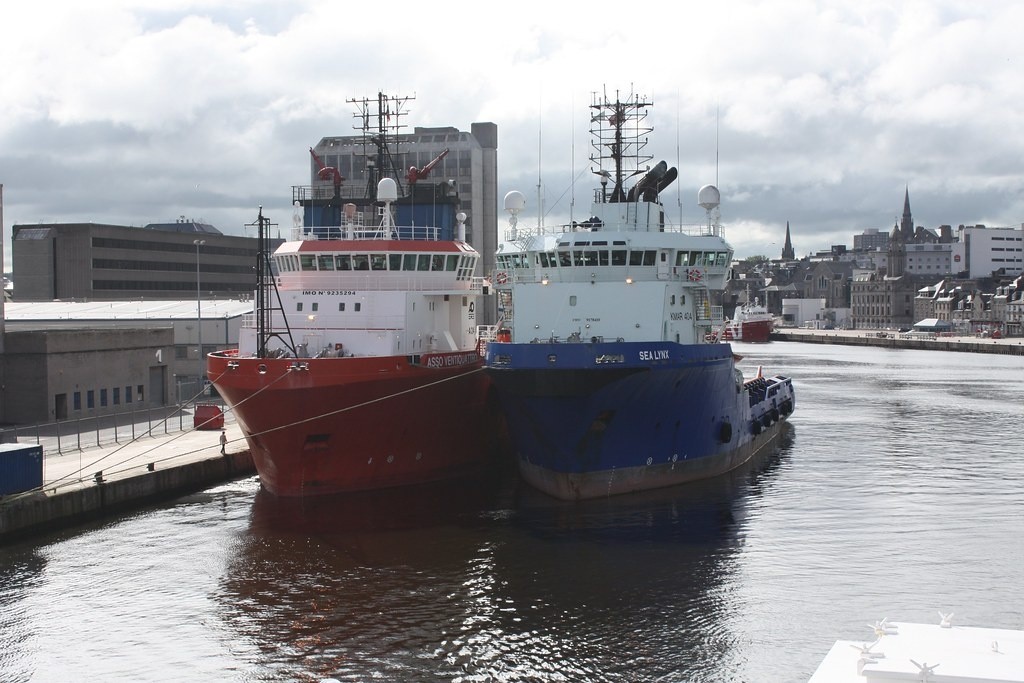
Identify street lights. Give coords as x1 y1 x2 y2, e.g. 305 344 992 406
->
194 239 205 407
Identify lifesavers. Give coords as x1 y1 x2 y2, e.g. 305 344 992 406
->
497 273 507 283
690 270 701 281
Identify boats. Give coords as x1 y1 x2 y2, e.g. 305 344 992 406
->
479 84 797 508
202 93 513 505
707 296 773 344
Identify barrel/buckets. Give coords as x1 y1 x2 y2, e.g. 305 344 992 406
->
497 329 510 342
